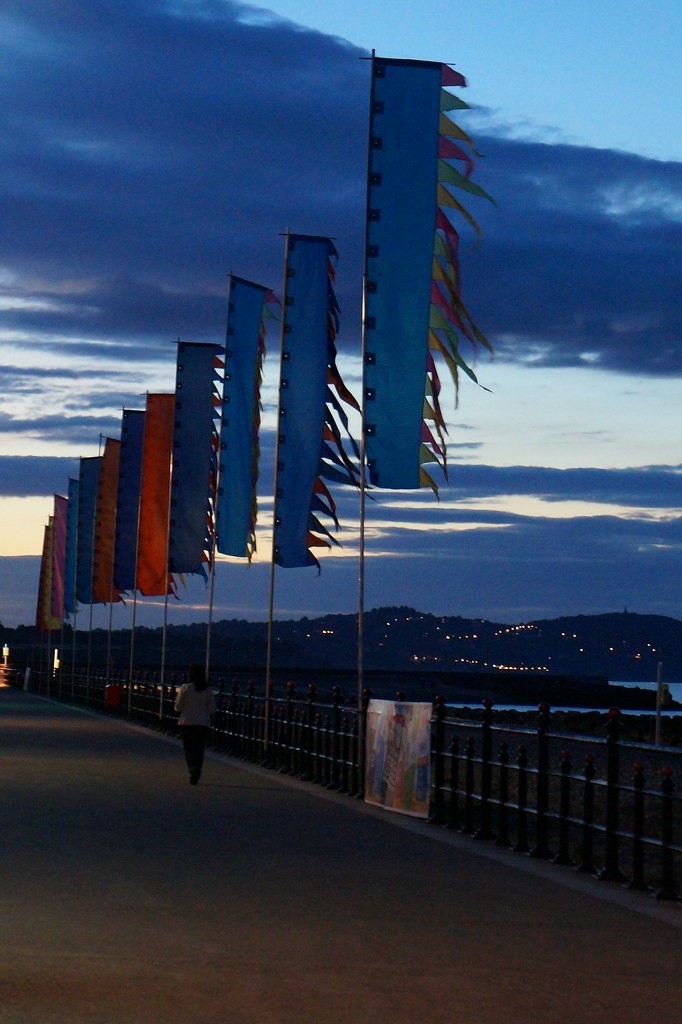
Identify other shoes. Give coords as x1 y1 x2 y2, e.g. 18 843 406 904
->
190 775 199 784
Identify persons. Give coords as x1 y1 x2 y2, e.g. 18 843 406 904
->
174 661 217 785
